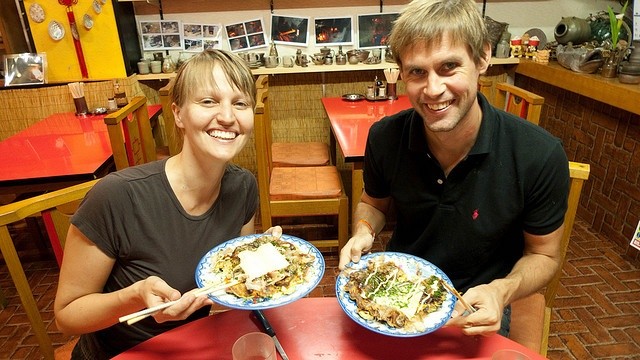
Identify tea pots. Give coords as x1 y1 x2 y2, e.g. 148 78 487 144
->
309 53 325 65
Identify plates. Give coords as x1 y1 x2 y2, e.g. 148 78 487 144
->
88 106 107 114
342 93 365 101
118 279 239 326
335 251 456 338
194 233 326 311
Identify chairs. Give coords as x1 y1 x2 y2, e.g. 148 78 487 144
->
154 76 181 166
253 89 350 257
495 84 545 126
44 95 159 273
257 74 333 167
0 178 102 359
508 162 591 359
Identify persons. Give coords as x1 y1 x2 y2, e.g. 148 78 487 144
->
54 49 258 359
337 0 569 338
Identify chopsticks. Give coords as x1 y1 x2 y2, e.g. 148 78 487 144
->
68 80 85 99
441 281 477 314
384 67 400 83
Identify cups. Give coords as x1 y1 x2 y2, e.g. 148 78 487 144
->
283 55 296 67
139 62 151 75
321 46 335 57
232 332 276 360
107 97 117 112
366 85 374 98
379 85 386 98
140 57 151 70
264 56 281 68
244 51 260 62
150 61 162 74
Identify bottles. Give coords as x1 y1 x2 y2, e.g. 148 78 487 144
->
336 45 346 65
373 75 379 97
375 81 383 97
269 39 279 66
295 49 302 65
115 91 128 107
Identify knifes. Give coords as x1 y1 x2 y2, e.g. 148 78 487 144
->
253 310 287 360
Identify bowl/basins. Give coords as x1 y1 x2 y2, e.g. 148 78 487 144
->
347 51 370 62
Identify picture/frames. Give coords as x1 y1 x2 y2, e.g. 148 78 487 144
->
3 53 45 86
